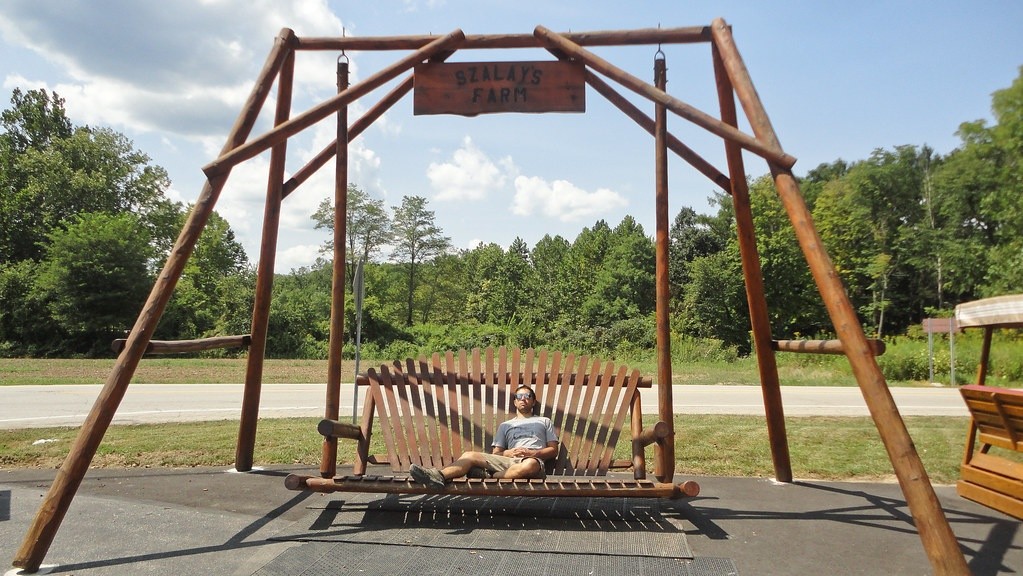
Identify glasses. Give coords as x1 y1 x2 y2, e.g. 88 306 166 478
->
513 393 533 400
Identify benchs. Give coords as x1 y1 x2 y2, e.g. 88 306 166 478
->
952 380 1023 522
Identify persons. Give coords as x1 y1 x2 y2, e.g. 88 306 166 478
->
408 385 560 496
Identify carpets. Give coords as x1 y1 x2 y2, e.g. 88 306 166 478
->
233 488 742 575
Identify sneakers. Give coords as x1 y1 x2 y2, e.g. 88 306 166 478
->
409 464 445 491
467 465 492 480
